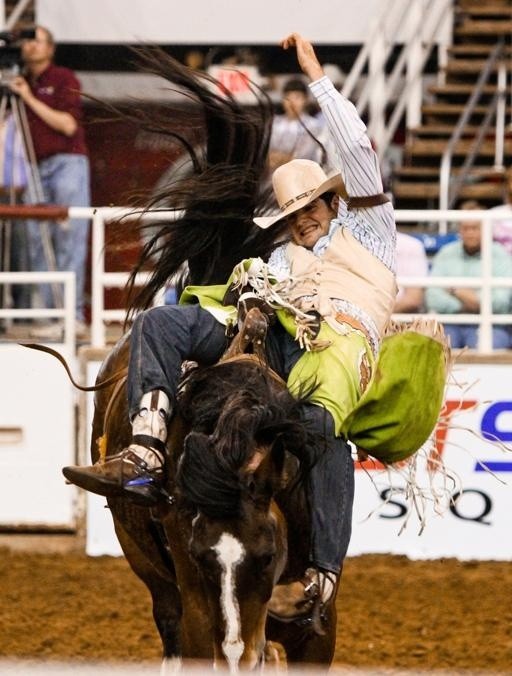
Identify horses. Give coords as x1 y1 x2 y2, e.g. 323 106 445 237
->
56 37 353 676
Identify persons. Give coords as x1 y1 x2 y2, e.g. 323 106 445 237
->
59 29 396 624
392 227 430 316
252 77 341 210
0 24 94 341
484 167 512 262
422 199 511 349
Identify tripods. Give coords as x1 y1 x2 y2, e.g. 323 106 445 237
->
0 73 65 334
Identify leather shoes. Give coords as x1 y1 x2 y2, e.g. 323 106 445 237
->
61 455 169 510
268 567 340 626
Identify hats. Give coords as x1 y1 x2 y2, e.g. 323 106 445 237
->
252 158 349 230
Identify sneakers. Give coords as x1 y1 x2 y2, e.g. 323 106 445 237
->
29 317 88 339
